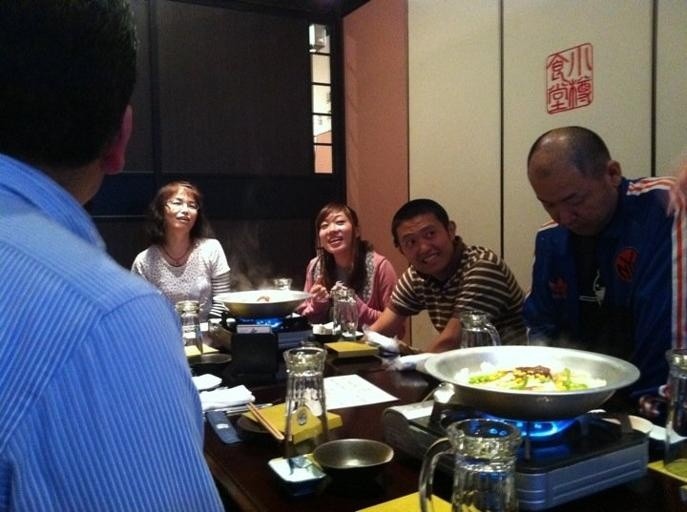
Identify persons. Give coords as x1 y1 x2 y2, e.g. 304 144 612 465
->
523 125 686 429
0 1 226 512
293 203 407 341
131 180 231 322
370 198 530 352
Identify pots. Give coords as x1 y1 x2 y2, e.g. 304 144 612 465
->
213 290 313 319
424 345 640 421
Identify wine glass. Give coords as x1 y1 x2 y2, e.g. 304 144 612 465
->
343 303 359 342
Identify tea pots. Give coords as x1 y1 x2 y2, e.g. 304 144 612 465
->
663 347 687 478
419 419 523 512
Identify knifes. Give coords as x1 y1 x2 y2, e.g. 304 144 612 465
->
202 403 273 415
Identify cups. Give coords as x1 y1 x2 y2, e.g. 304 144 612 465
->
333 290 355 332
273 276 293 290
174 299 205 357
283 347 329 468
458 310 502 347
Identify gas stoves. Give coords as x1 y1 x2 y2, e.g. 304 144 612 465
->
380 382 649 512
208 311 315 352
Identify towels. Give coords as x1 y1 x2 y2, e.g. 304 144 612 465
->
199 385 256 412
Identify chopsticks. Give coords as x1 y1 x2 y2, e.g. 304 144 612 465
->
246 402 285 440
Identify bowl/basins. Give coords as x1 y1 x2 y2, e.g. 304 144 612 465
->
311 330 342 347
312 438 395 479
188 354 232 371
